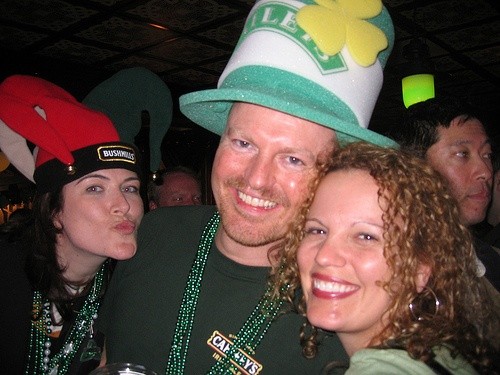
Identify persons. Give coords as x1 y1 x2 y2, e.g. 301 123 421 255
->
0 128 145 375
267 99 499 375
148 166 203 211
97 0 410 375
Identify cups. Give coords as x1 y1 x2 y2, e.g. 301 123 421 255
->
88 363 160 375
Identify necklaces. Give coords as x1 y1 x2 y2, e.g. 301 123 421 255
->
163 207 289 375
23 258 110 375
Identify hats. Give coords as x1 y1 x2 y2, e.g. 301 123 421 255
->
0 75 136 190
180 0 400 150
